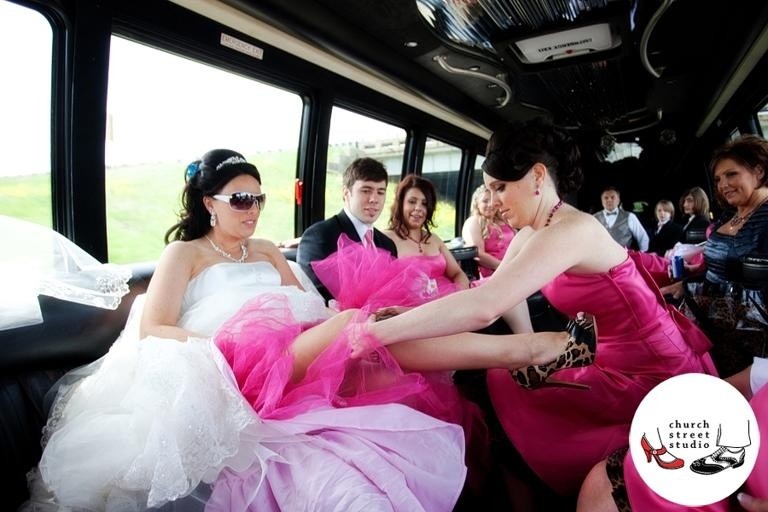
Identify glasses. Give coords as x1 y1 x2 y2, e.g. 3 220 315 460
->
213 192 266 212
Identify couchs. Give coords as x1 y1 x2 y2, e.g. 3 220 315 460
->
0 260 161 511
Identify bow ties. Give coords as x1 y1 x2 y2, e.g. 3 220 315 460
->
607 212 617 216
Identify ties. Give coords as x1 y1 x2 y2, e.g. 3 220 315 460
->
365 230 374 251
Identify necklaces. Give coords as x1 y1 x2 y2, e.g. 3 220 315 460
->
729 196 765 228
541 201 563 227
203 235 251 263
406 233 430 253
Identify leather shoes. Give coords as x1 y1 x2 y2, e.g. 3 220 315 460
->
691 448 746 475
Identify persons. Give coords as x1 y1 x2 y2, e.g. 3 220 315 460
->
594 188 650 254
651 201 683 257
660 137 767 375
28 149 597 512
622 213 719 282
379 175 532 334
296 156 397 308
463 184 522 278
573 355 767 512
351 113 720 512
684 186 717 249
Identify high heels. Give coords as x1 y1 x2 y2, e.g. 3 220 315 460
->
509 311 597 392
642 430 684 472
